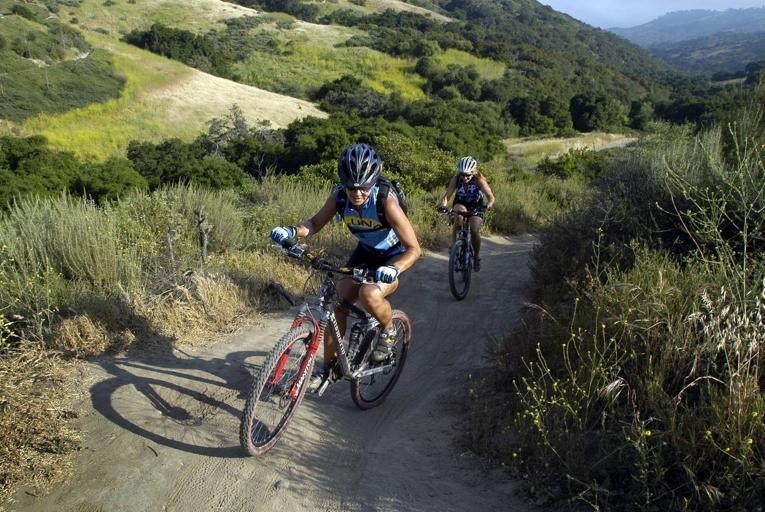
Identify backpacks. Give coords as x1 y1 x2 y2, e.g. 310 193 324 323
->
378 176 407 216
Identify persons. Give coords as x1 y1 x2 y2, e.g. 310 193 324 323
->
270 142 422 394
438 155 495 272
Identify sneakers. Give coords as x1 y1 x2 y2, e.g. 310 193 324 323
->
308 372 328 393
371 322 398 362
474 258 480 272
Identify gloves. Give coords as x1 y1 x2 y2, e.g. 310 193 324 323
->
271 226 297 244
376 265 399 283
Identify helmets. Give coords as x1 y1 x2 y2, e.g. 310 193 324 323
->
338 143 381 189
458 156 476 174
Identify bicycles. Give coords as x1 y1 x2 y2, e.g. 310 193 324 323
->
439 207 488 301
240 228 411 457
